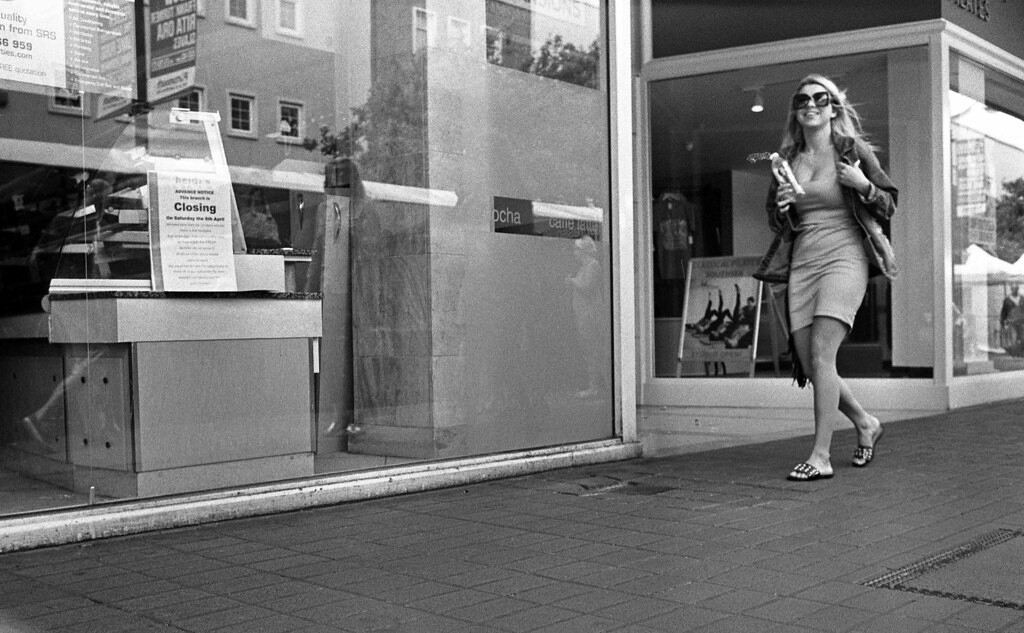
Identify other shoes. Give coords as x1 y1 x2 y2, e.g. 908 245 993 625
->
23 415 56 450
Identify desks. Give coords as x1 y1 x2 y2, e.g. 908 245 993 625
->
0 300 321 501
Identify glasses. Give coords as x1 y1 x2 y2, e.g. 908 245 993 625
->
792 92 832 110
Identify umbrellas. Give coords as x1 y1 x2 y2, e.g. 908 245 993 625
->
953 245 1024 296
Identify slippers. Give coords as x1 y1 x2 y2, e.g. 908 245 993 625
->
852 425 883 467
786 463 834 480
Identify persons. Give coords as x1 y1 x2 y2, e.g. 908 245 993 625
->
653 168 704 318
304 159 380 437
32 180 126 279
1000 284 1024 358
766 73 900 481
685 283 756 350
570 234 612 399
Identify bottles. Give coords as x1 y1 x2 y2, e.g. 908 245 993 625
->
769 153 805 203
